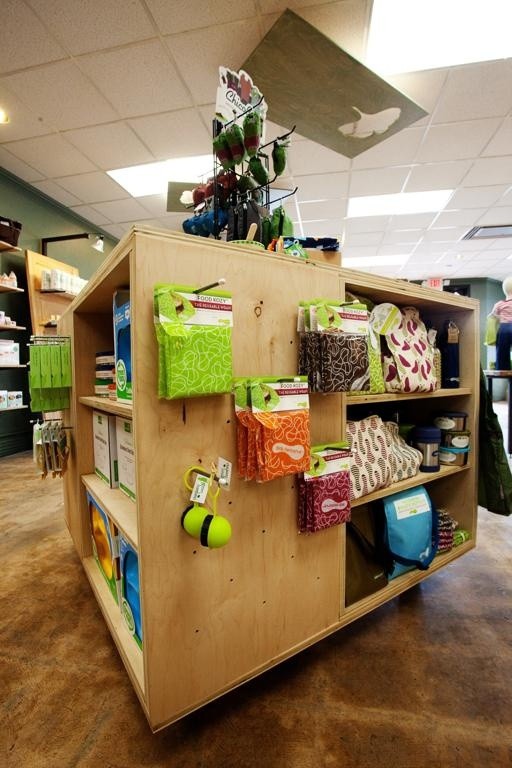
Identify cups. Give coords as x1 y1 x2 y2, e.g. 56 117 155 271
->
405 425 441 472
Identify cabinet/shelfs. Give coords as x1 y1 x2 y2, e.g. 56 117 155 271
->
35 289 76 327
0 241 28 411
71 227 339 734
344 279 480 615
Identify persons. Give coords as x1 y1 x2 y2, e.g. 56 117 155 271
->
491 278 512 370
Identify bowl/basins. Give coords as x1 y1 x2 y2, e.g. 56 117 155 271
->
228 239 265 249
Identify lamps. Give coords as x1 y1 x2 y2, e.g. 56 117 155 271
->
92 234 104 253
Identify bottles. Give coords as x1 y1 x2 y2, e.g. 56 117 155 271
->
399 425 416 440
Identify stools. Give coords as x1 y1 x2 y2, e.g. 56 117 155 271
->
482 369 512 458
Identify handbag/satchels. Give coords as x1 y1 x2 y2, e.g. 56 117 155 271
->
478 366 512 517
345 291 385 397
345 415 425 499
345 501 388 608
380 305 437 392
369 485 435 580
